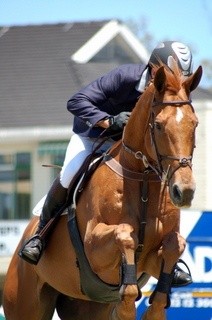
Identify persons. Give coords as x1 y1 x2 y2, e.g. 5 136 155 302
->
22 41 192 288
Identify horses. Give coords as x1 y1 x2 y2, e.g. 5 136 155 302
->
2 57 203 320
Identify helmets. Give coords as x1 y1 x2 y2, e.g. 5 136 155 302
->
147 41 193 77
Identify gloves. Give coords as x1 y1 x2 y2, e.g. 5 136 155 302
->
110 111 130 129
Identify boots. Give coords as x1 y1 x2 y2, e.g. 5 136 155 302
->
159 259 193 289
16 175 70 266
